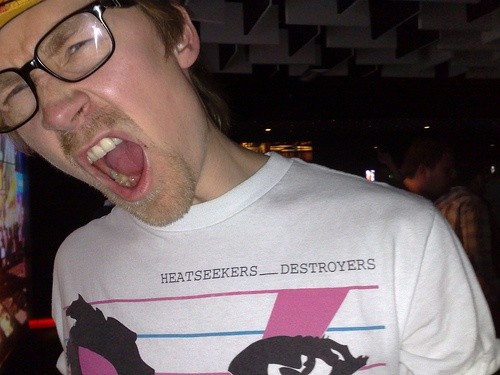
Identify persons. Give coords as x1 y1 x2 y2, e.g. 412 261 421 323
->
0 0 497 375
389 138 454 199
431 150 497 300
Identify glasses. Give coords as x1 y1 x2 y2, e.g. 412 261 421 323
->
0 0 140 134
